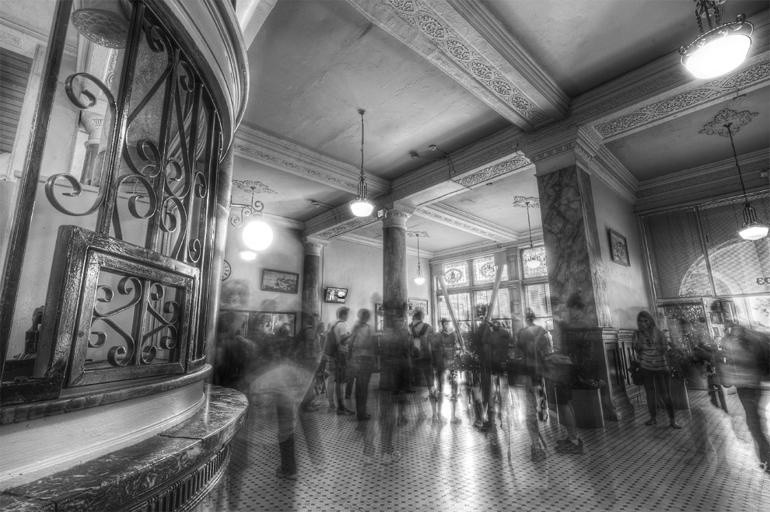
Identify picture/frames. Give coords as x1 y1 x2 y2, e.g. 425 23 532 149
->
261 268 299 294
324 286 349 304
605 227 632 267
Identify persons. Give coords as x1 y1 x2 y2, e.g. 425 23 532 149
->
216 301 770 512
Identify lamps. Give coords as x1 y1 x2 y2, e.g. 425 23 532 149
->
345 108 376 218
720 120 769 245
667 1 759 83
521 198 544 271
412 230 428 288
